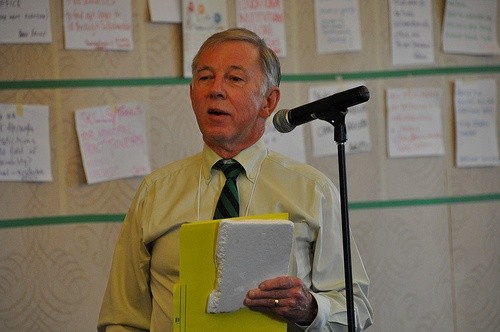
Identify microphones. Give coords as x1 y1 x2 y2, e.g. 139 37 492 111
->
273 85 370 133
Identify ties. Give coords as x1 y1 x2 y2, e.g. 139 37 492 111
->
210 160 245 220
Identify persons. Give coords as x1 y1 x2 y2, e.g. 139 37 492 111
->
272 298 279 307
97 28 374 332
